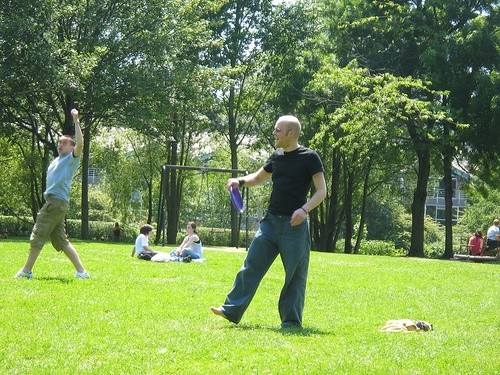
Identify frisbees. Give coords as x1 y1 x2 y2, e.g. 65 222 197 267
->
230 186 243 213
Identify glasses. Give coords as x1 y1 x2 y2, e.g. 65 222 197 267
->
273 127 280 132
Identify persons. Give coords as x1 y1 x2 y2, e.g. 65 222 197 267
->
12 109 89 280
173 222 203 262
487 219 500 257
132 225 157 260
112 223 121 242
211 115 327 327
468 231 483 255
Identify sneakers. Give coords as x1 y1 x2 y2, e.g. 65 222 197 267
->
15 270 34 278
416 321 431 331
183 255 191 262
74 271 90 279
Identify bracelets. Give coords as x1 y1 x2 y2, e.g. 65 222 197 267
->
302 206 311 214
237 176 245 187
